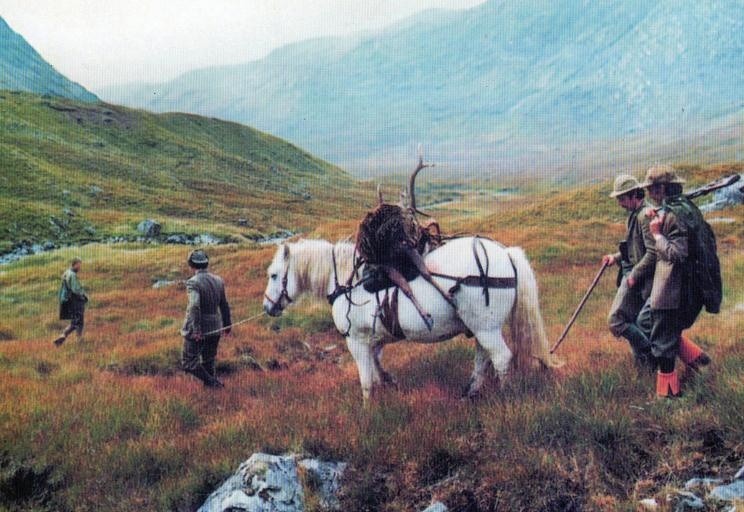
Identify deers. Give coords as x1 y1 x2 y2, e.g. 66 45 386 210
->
354 142 458 333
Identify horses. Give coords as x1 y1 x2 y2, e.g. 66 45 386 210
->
260 236 551 410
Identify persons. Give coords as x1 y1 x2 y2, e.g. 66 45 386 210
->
179 250 234 389
53 258 89 347
602 175 660 371
642 165 722 401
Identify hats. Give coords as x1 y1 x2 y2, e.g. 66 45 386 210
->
609 175 639 198
638 164 677 189
187 247 209 265
